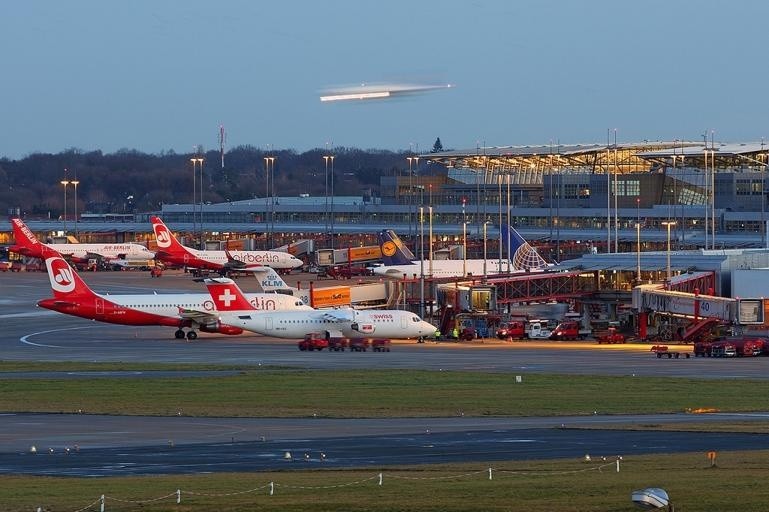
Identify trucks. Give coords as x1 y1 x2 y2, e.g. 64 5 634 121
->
496 319 625 344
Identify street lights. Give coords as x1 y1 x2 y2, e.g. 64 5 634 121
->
670 128 716 250
605 128 620 253
474 136 487 242
546 137 561 263
406 142 420 258
661 200 676 277
757 137 769 248
323 141 334 248
61 169 79 238
190 145 204 250
264 144 275 249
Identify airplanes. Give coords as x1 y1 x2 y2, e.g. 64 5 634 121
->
149 214 303 279
364 223 569 280
8 217 155 271
35 242 315 341
176 261 442 354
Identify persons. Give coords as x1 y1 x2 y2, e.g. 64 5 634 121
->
452 328 458 341
435 328 441 342
